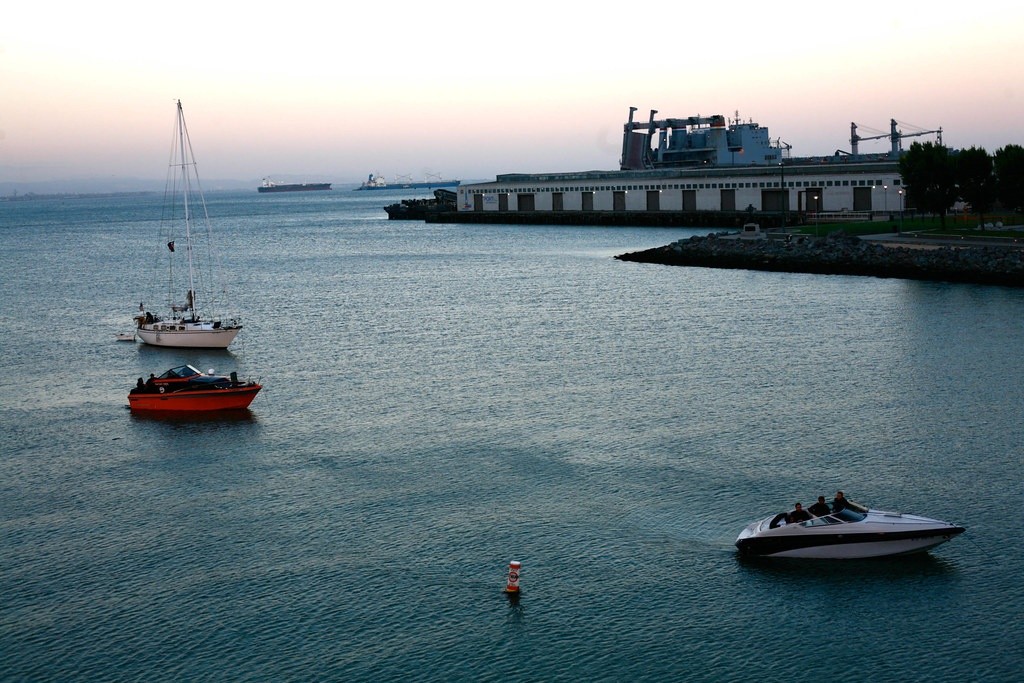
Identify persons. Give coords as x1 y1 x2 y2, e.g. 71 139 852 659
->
146 312 154 324
790 503 810 523
833 491 850 512
146 373 159 385
809 496 831 518
137 378 146 389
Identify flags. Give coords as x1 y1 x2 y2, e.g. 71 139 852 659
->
167 241 174 252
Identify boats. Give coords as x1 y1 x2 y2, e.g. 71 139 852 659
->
135 96 243 350
735 499 968 560
128 364 263 412
